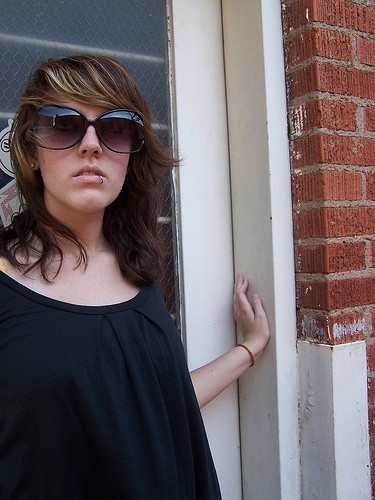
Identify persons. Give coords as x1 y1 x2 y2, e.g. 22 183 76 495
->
0 53 271 500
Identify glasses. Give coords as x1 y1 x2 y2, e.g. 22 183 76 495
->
32 104 145 154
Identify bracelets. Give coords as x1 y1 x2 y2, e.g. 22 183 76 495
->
236 344 255 368
